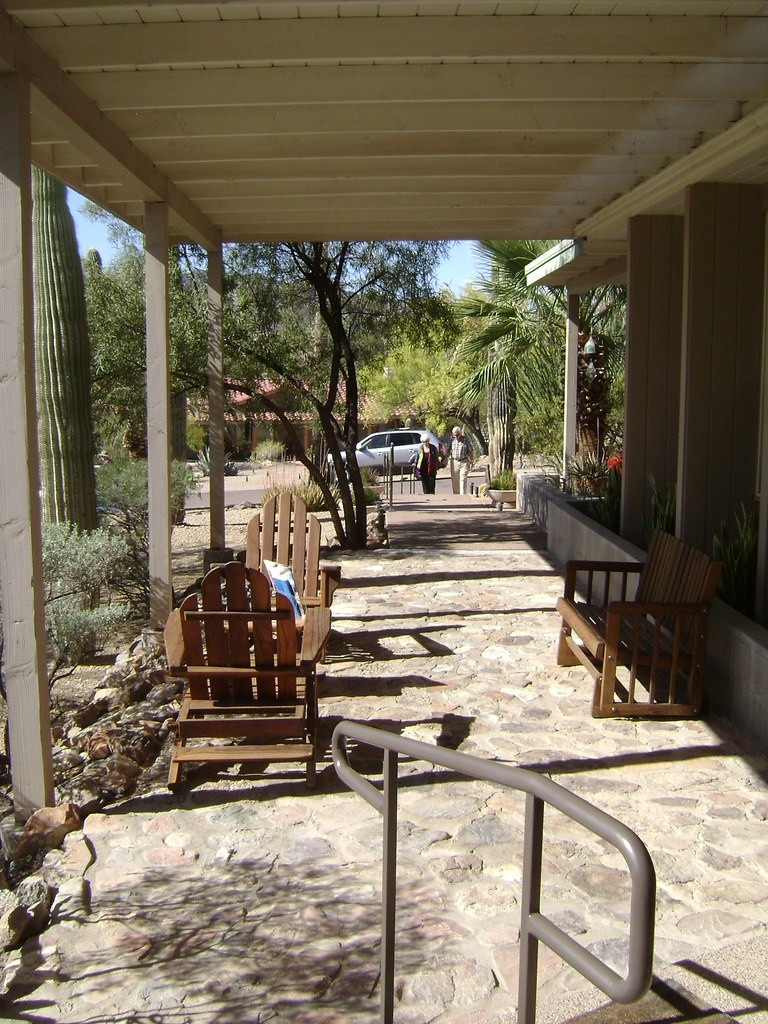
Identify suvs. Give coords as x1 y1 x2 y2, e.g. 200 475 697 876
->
324 427 448 481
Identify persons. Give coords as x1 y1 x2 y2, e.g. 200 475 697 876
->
445 427 473 495
412 434 441 495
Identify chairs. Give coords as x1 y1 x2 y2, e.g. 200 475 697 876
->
556 531 724 718
163 559 333 793
210 490 342 663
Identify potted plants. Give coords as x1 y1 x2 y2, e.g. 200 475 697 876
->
567 447 611 496
485 470 517 512
360 466 385 494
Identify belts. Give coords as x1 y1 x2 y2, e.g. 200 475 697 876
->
452 456 467 461
